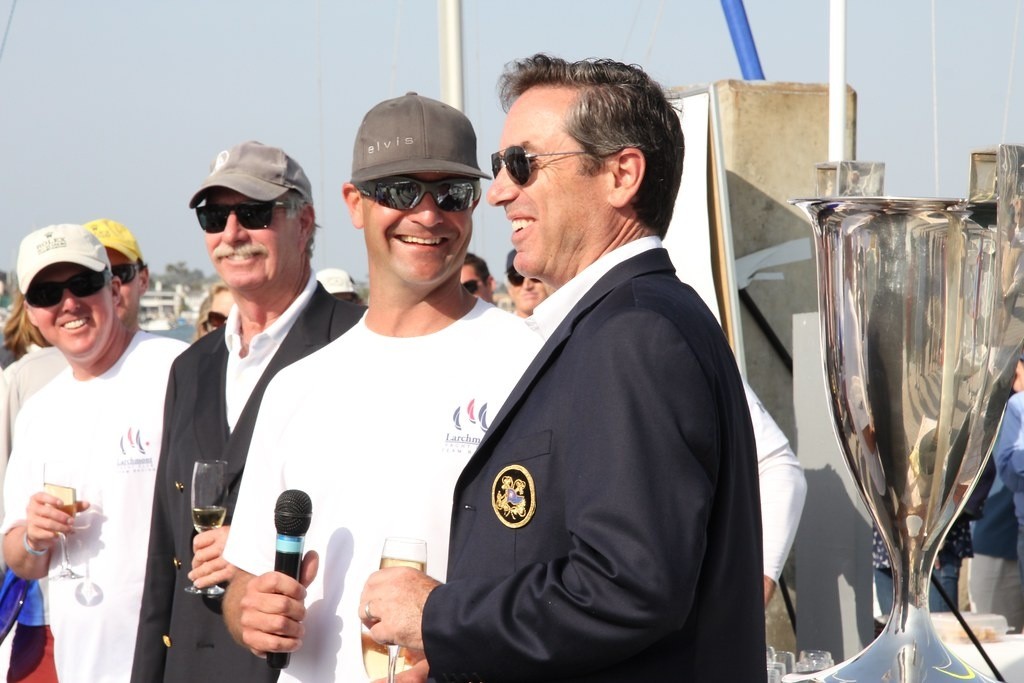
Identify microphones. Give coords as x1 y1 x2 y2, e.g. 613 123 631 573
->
266 489 312 667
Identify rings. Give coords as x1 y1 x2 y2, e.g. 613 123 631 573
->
365 602 377 622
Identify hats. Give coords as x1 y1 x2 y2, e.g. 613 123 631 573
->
189 140 313 211
506 250 517 271
350 92 493 186
17 223 112 295
82 219 143 266
315 268 362 300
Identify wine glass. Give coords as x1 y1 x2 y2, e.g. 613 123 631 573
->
183 458 229 597
45 460 80 580
380 536 428 683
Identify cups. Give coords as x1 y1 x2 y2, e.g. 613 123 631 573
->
766 647 833 683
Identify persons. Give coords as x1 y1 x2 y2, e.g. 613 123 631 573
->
741 376 808 608
197 284 235 339
359 49 769 683
506 248 549 317
461 253 495 303
968 475 1024 634
316 268 364 304
991 350 1024 577
0 217 191 683
130 138 370 683
222 91 525 683
871 448 998 615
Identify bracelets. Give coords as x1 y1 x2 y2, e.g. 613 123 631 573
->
23 532 48 556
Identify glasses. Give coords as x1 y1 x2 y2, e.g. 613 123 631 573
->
110 263 143 284
206 311 228 328
355 176 482 212
25 268 111 308
195 200 296 234
464 277 488 294
490 146 595 186
507 271 541 287
